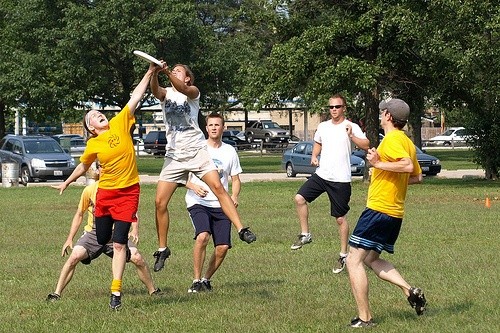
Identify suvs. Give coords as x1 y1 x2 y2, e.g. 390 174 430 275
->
0 133 77 187
223 130 250 149
144 131 168 156
428 127 484 147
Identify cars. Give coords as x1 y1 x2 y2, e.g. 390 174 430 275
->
245 122 290 143
281 141 365 178
415 147 442 177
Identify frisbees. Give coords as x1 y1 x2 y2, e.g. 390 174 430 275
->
133 49 164 68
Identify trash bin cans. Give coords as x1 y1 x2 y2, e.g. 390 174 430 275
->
2 162 19 188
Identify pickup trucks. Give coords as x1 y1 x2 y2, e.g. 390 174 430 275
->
53 135 88 159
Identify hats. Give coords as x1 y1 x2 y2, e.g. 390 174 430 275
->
381 99 410 122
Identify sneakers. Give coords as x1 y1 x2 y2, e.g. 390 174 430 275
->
290 234 312 250
153 247 171 272
333 255 347 273
347 316 376 328
201 280 213 294
148 288 164 298
109 293 121 309
188 281 201 293
407 287 426 317
238 227 256 244
46 293 61 302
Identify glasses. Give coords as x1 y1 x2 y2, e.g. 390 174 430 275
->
97 164 102 168
330 105 344 109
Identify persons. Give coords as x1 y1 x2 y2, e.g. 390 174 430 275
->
344 96 429 329
49 61 159 309
45 159 162 302
148 59 257 272
289 93 371 274
185 110 243 293
